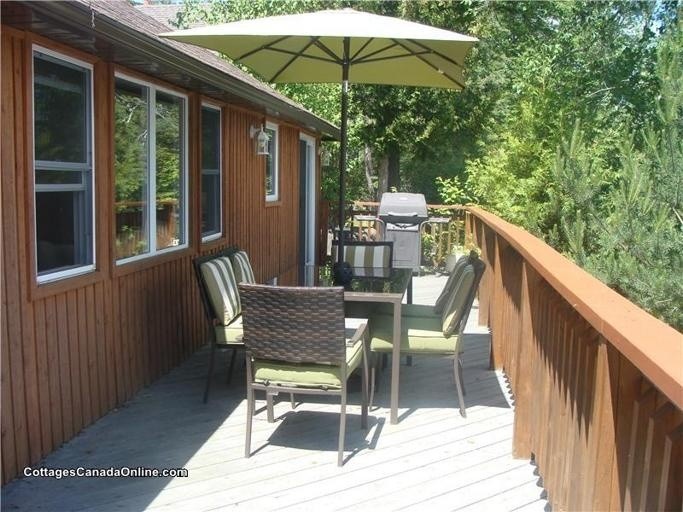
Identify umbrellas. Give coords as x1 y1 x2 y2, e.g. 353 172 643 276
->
158 9 482 284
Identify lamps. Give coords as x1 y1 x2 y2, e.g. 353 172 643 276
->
248 123 270 156
319 144 332 167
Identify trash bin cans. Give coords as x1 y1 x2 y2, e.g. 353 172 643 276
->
379 191 428 276
